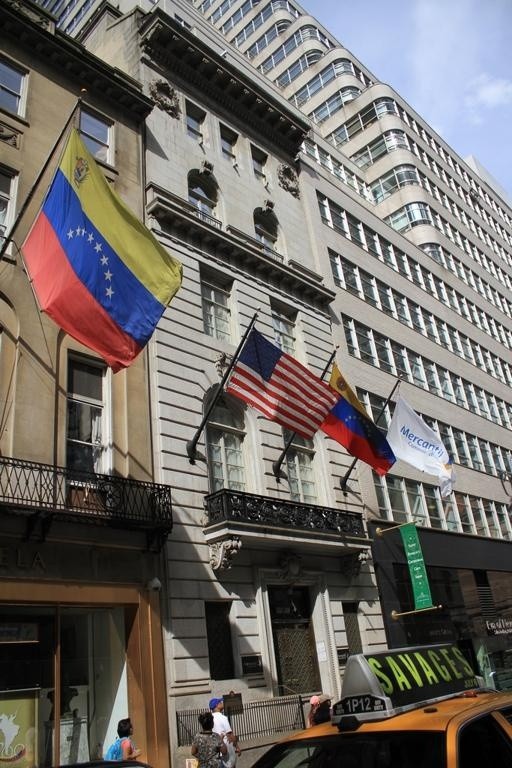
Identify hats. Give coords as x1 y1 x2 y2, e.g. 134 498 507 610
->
209 698 224 709
319 694 333 703
310 695 319 705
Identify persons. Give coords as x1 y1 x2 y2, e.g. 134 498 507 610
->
307 694 335 728
104 717 142 761
191 697 241 768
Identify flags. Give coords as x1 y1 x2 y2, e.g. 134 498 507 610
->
23 127 185 374
222 326 341 440
320 361 397 478
385 397 453 499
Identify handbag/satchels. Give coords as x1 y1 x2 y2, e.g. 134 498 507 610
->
221 743 237 768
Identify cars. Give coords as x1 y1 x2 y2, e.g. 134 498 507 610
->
57 761 150 768
250 643 512 768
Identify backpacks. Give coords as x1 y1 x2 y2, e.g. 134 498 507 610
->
104 738 136 761
